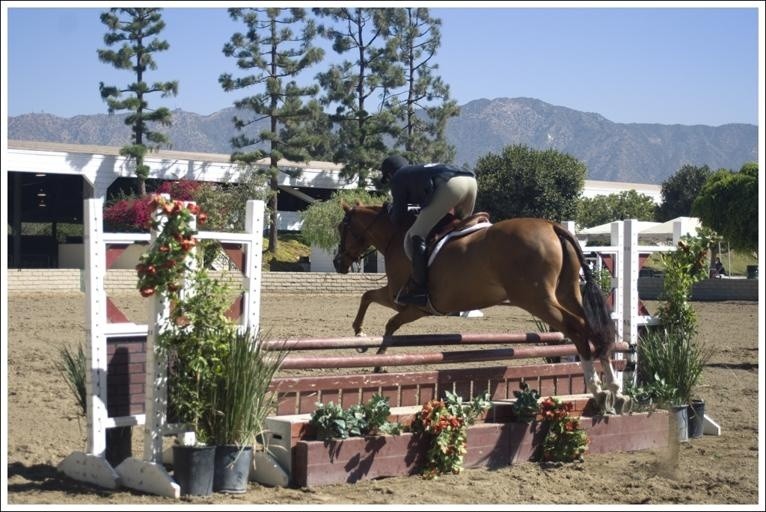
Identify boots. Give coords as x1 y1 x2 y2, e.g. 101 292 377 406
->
393 235 428 306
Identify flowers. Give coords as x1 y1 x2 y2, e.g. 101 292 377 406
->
133 189 209 297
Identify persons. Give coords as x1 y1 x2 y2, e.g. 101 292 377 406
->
380 154 478 306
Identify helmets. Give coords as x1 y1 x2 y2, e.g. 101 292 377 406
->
380 155 407 184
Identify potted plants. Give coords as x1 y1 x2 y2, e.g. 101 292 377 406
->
212 322 299 493
638 325 714 441
156 269 231 498
650 227 722 439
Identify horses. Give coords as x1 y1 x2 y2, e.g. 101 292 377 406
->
332 197 634 415
